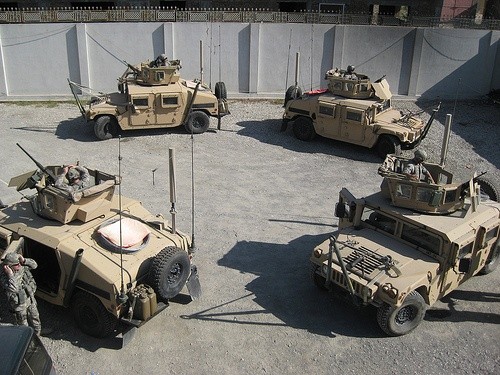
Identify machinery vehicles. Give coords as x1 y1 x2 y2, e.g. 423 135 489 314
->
68 38 231 140
307 113 499 336
279 51 443 160
1 143 204 338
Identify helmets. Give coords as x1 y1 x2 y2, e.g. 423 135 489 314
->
3 253 20 265
347 64 355 71
414 150 427 161
159 54 167 62
66 169 80 180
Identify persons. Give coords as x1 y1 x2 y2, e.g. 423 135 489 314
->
0 253 42 340
151 54 171 68
55 164 91 193
343 65 358 81
403 148 435 183
